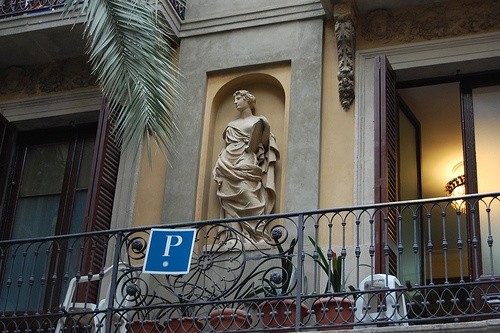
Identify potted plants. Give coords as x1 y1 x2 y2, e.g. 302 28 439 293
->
173 267 266 333
304 235 359 329
159 278 203 333
116 269 165 333
241 236 309 333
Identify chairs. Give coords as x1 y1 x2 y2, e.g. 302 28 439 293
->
53 262 133 333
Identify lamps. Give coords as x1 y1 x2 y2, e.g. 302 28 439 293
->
445 161 467 214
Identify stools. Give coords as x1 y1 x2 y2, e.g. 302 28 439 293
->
353 274 409 329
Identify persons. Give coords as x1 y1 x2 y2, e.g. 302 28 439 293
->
211 89 280 246
